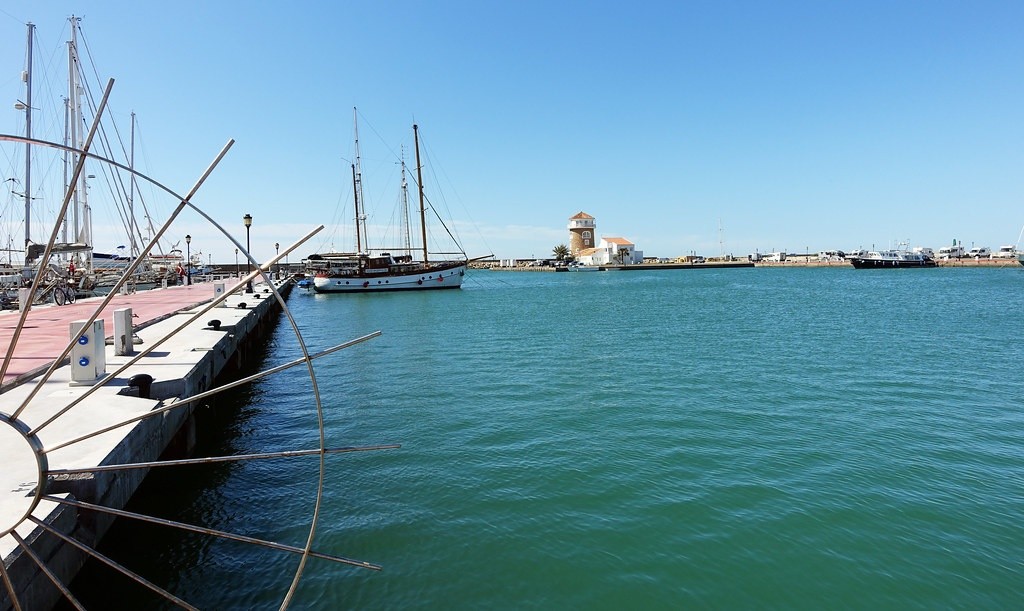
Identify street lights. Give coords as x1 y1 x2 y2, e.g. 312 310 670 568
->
275 242 280 280
235 248 240 278
243 213 254 293
185 235 194 285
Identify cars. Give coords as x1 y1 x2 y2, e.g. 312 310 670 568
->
693 258 705 263
530 261 584 267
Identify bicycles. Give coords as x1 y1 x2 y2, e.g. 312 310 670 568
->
53 274 76 306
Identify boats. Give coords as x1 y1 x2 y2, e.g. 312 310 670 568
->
848 251 940 268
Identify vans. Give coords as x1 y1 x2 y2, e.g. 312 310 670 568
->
761 250 848 263
913 247 935 258
940 247 965 259
999 245 1017 259
969 248 991 258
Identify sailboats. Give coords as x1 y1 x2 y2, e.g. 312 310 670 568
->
0 14 225 312
311 106 497 293
